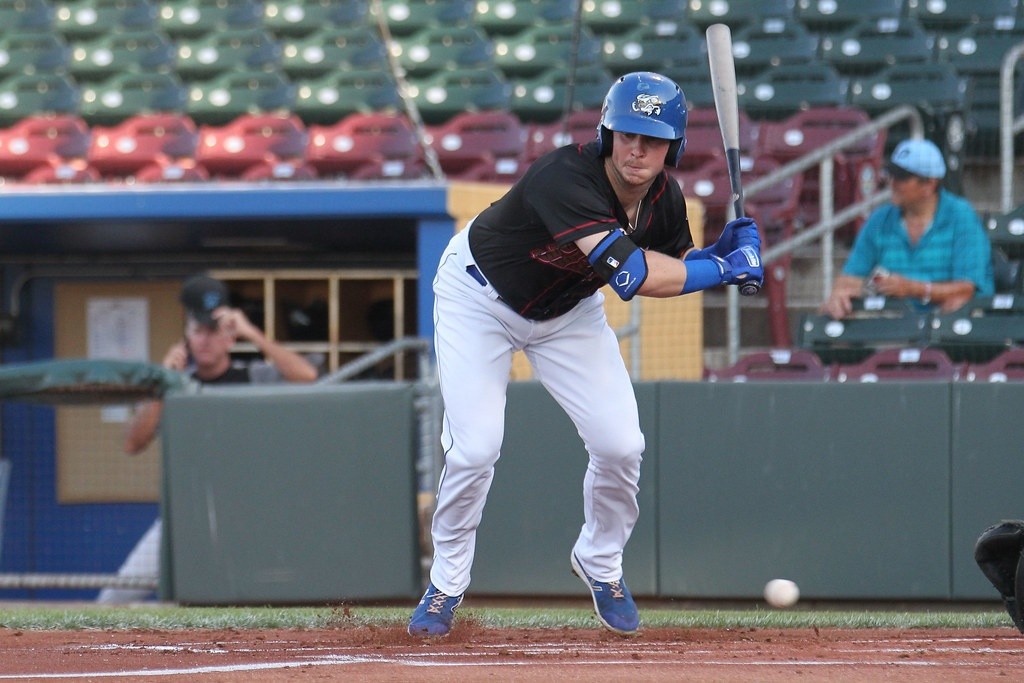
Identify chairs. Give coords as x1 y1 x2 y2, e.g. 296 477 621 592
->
1 0 1024 181
717 197 1024 386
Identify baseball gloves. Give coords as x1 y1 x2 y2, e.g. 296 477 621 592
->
974 518 1024 636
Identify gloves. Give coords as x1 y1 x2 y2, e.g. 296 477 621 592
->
711 217 762 290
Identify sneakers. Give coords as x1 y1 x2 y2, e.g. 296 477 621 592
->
406 582 464 640
569 545 639 637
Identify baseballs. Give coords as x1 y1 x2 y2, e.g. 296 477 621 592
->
762 579 801 609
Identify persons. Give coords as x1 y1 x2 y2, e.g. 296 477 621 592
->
407 73 763 642
826 140 995 320
92 280 317 604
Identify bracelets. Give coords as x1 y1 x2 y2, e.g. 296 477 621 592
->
922 282 932 305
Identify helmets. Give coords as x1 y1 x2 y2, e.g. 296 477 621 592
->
595 71 689 168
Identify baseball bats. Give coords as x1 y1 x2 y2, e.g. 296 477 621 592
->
706 24 762 297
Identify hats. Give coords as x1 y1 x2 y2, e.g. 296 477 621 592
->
880 138 946 182
182 277 228 329
